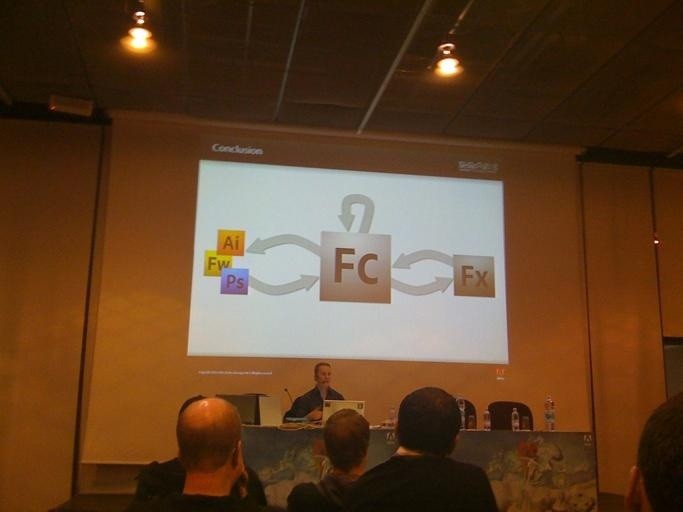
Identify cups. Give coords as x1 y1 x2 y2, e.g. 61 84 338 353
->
522 416 531 430
468 416 477 432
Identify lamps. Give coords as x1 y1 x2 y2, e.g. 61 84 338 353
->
434 40 460 69
126 8 152 39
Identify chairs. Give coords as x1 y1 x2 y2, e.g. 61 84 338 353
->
457 398 480 430
487 399 533 427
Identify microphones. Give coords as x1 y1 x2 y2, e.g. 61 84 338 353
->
284 387 307 423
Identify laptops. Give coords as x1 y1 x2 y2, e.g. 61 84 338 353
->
322 400 366 424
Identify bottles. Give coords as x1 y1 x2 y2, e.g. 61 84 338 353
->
544 395 556 431
456 392 466 430
483 409 491 431
389 403 397 430
511 408 520 431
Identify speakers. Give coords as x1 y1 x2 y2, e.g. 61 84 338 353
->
49 95 96 117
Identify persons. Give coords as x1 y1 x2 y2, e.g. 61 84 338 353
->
624 393 683 512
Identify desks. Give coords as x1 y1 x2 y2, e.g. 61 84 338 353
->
206 426 598 512
